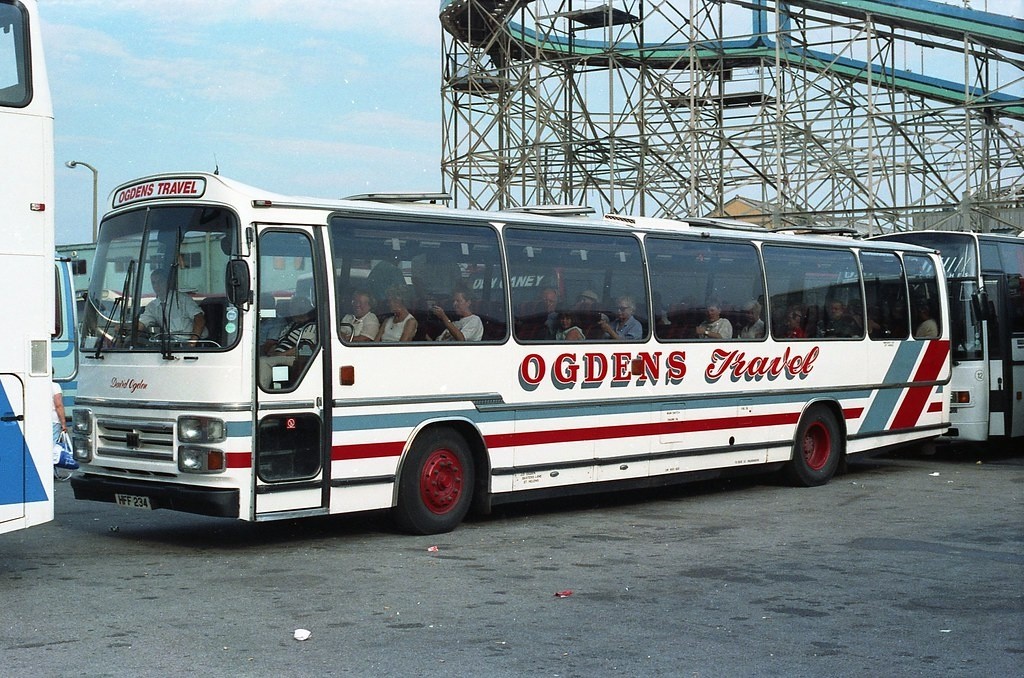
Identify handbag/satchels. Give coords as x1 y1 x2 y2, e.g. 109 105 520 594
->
53 431 79 469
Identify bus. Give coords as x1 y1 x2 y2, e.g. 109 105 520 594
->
1 1 54 535
852 231 1023 446
56 252 80 470
68 168 955 535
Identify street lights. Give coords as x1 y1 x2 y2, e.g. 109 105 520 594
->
64 160 98 244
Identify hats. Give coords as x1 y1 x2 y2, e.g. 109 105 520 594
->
581 291 598 299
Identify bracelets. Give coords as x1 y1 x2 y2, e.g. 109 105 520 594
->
446 320 450 328
704 328 710 335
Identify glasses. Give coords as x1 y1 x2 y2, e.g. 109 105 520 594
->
617 307 631 311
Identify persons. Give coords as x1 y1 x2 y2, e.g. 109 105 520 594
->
52 367 67 480
433 285 483 341
740 299 766 339
544 287 598 340
876 304 907 337
114 269 205 347
375 289 417 342
368 244 463 338
598 295 642 340
773 304 808 339
259 293 316 389
825 300 852 338
340 293 380 341
696 297 732 339
916 303 938 337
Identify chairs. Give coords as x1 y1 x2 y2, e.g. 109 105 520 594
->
199 296 229 347
377 283 819 343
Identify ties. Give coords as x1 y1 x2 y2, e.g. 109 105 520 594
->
162 302 168 331
